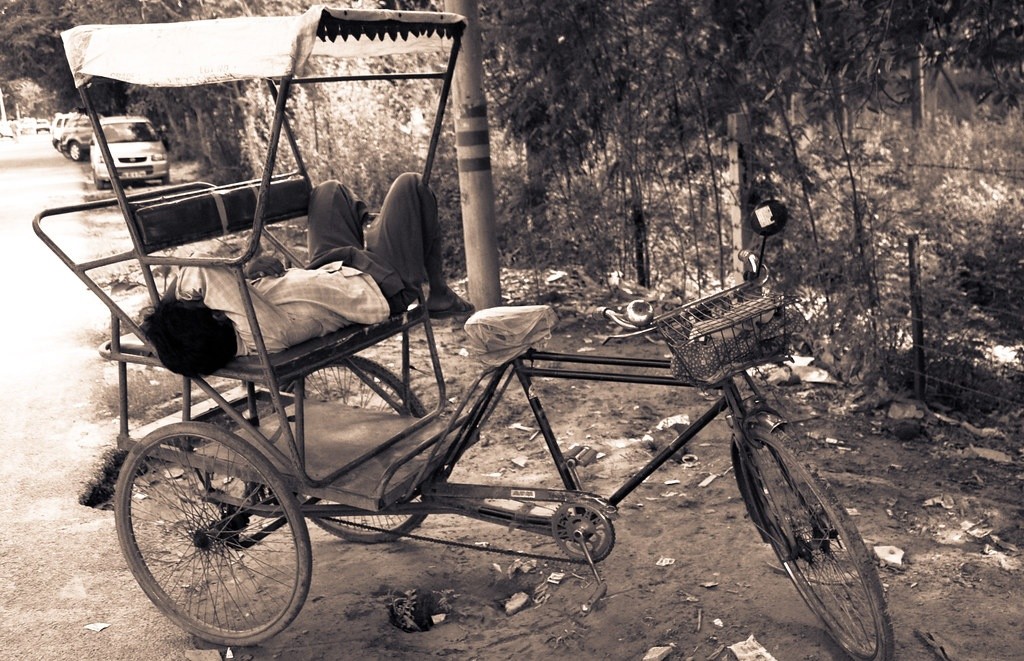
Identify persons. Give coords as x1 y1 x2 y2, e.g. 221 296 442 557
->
139 172 476 378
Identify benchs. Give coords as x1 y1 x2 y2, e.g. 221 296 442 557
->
109 171 445 487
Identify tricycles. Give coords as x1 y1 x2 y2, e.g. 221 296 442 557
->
28 7 897 661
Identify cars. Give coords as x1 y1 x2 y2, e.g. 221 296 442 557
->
87 116 175 190
0 108 101 161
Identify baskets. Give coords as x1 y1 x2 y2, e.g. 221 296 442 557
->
655 282 803 390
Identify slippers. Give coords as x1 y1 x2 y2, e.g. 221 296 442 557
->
427 292 476 318
